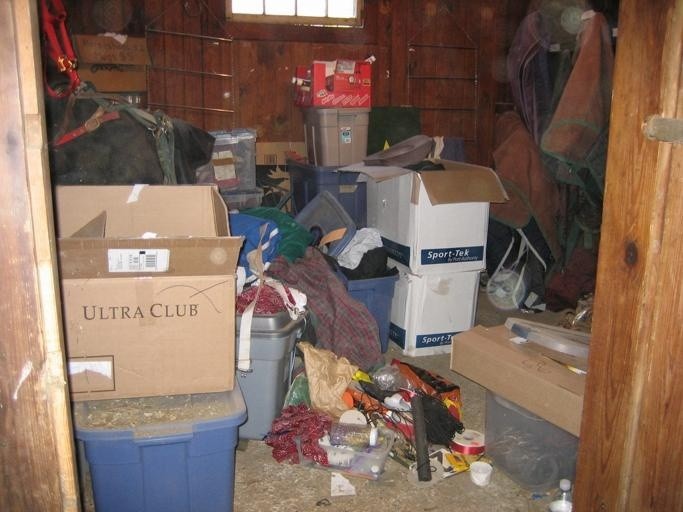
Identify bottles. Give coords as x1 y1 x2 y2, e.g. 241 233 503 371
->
549 479 573 512
331 422 377 448
291 77 311 88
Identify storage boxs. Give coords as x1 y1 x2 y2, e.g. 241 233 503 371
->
483 388 581 493
54 183 248 404
209 128 257 194
347 273 401 355
68 34 151 96
292 59 374 109
385 255 480 359
296 426 396 478
449 322 590 440
303 105 374 170
337 157 510 276
221 184 265 213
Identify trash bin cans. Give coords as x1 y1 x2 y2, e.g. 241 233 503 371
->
336 265 399 353
298 106 371 167
